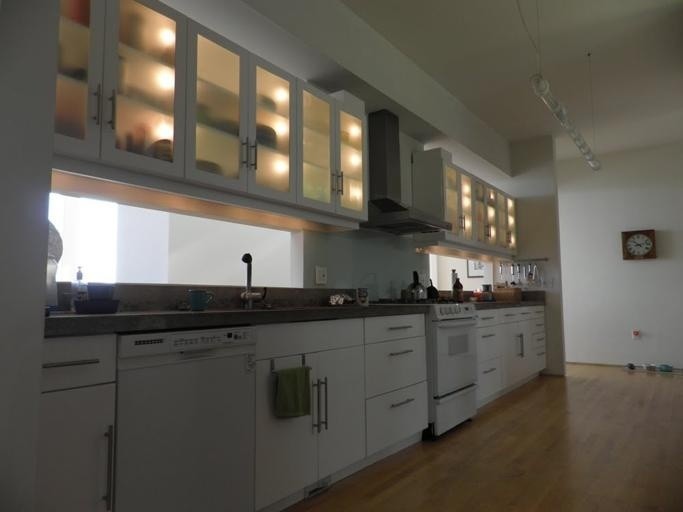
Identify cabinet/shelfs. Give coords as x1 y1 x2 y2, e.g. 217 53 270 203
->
473 178 497 251
474 307 500 413
296 81 367 220
50 0 182 183
252 317 368 510
502 306 531 393
530 305 546 375
497 191 517 254
443 159 477 247
36 331 119 509
183 15 298 209
363 314 428 470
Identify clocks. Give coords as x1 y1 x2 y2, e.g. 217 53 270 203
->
620 229 656 262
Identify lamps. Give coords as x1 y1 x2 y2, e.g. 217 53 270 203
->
528 1 605 176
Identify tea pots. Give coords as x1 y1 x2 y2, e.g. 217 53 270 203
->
426 277 442 301
404 270 428 302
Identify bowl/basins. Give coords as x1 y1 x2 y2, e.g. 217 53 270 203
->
643 363 656 371
660 364 673 372
87 284 115 300
73 298 119 314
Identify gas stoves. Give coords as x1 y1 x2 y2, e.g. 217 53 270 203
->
379 297 477 320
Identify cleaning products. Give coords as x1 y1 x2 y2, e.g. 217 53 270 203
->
451 269 458 298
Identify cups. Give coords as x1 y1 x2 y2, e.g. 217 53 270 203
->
482 284 494 301
355 287 369 305
187 288 215 312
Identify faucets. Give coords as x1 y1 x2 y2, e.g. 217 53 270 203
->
240 253 267 308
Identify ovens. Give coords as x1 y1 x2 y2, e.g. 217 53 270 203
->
428 304 479 437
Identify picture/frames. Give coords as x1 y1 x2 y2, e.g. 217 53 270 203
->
466 259 485 278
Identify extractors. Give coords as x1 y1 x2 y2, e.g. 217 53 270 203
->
359 109 452 236
